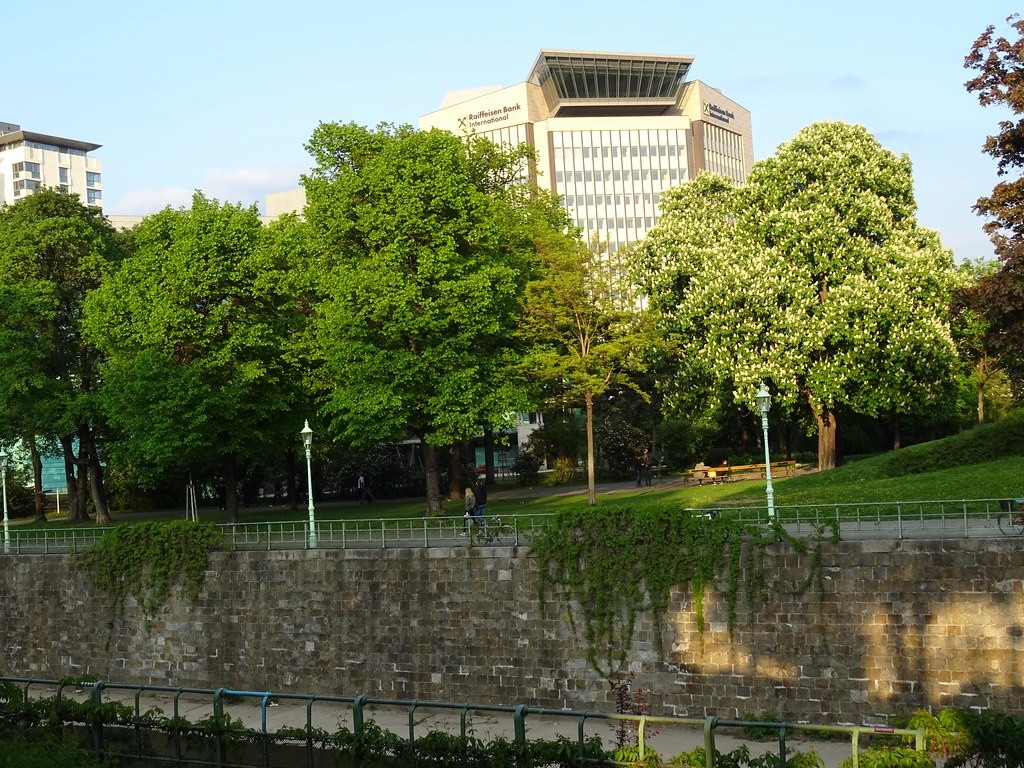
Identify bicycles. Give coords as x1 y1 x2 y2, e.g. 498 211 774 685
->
466 515 518 549
667 505 730 546
996 497 1024 538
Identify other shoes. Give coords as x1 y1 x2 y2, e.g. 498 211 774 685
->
460 531 466 536
477 530 482 536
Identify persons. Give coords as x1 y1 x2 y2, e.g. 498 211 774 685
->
269 475 308 508
635 449 651 487
1008 503 1024 525
460 478 487 536
214 476 252 512
694 458 729 487
358 471 377 504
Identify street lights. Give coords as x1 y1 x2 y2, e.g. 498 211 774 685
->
753 378 779 530
300 418 318 549
0 444 10 554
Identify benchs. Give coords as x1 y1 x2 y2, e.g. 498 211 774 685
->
685 461 797 486
